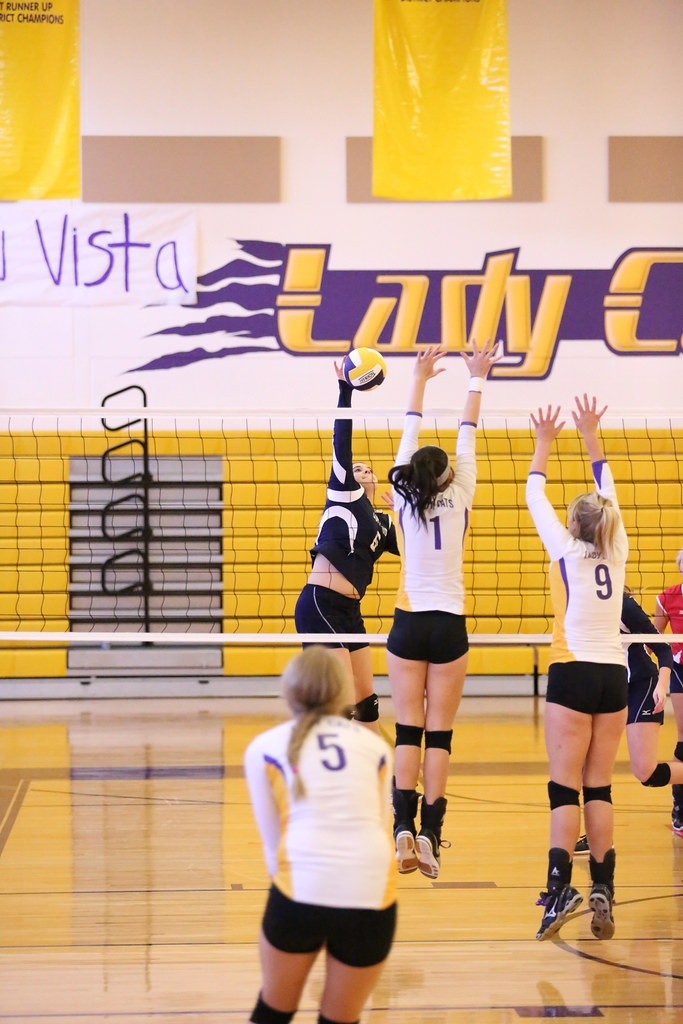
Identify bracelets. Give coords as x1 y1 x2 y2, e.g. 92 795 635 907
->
468 376 484 393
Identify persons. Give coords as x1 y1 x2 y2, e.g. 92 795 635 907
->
386 338 504 878
525 393 628 941
244 646 397 1024
295 355 400 739
573 549 683 854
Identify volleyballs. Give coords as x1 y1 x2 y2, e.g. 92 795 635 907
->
343 347 387 392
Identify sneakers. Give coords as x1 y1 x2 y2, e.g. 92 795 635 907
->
588 884 615 940
413 828 451 879
393 824 418 874
535 886 583 941
672 807 683 832
573 834 614 854
